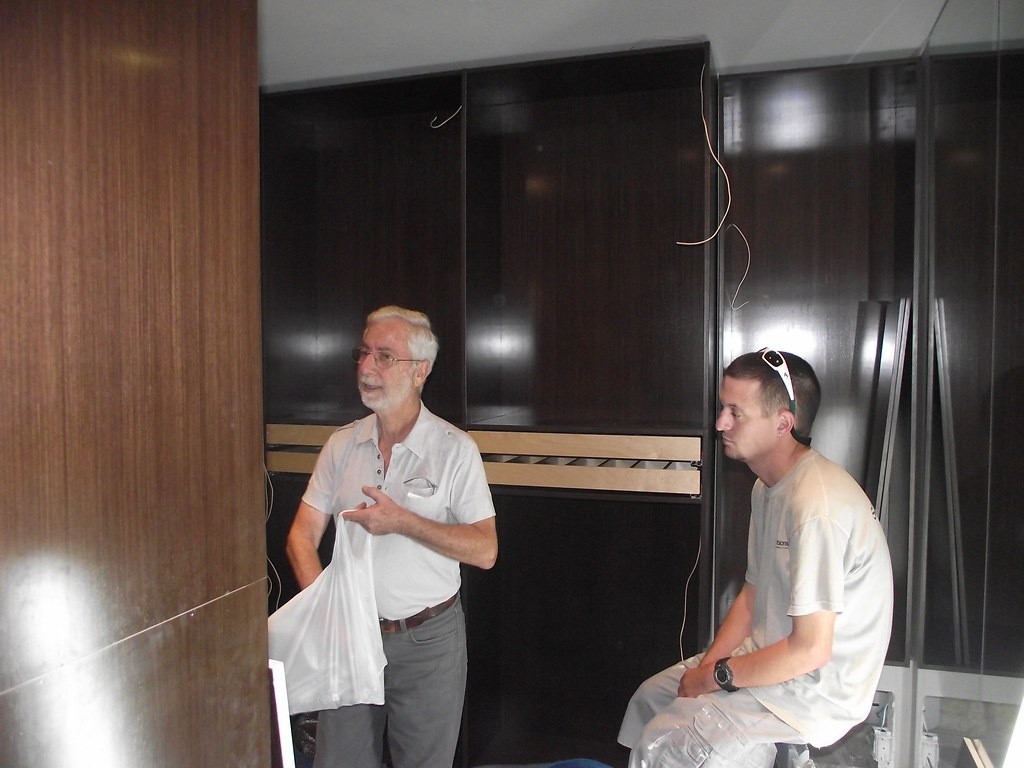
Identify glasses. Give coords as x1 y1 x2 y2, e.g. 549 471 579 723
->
351 346 420 369
758 347 796 418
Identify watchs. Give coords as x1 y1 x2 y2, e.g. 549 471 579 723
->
713 657 740 692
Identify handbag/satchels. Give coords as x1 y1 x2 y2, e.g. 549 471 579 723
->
267 509 388 715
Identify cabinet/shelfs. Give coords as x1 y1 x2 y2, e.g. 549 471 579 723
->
259 41 717 767
717 49 1023 767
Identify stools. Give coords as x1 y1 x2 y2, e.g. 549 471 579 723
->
773 723 875 768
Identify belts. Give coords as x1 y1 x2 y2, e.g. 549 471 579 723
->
379 592 458 634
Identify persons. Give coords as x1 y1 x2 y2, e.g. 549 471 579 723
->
616 351 894 768
286 306 498 768
